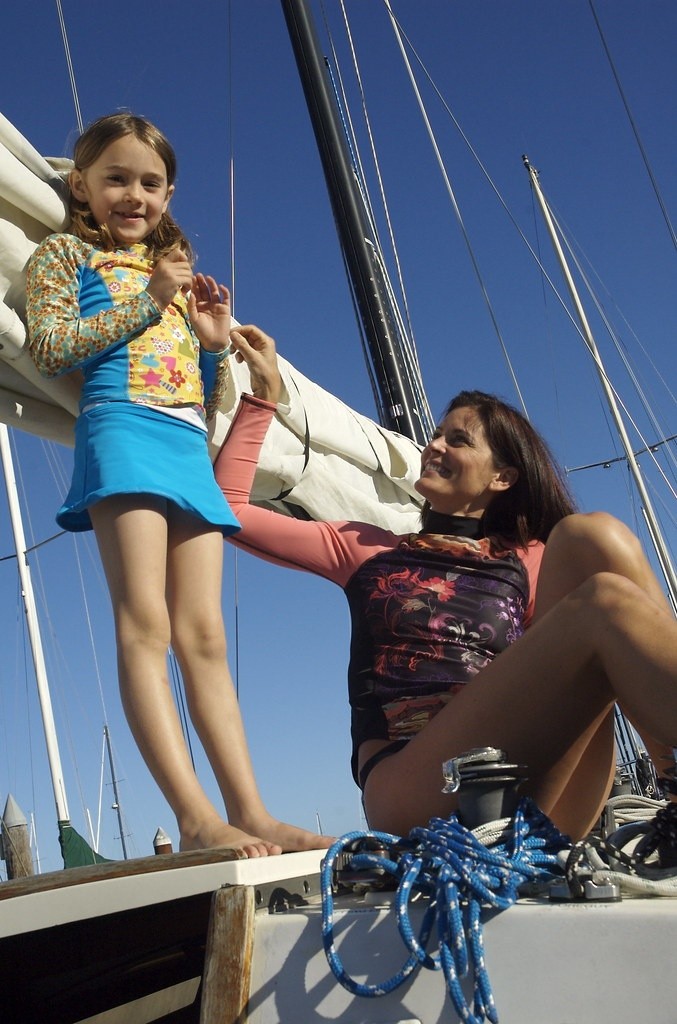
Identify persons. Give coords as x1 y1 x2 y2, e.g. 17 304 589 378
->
209 323 677 843
25 112 341 861
